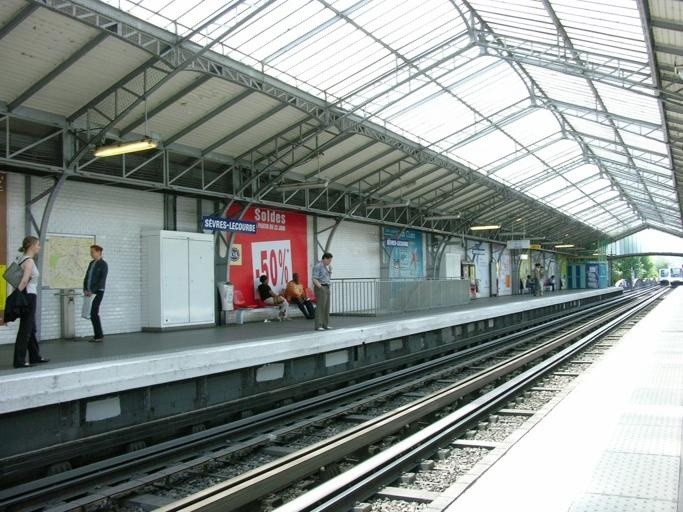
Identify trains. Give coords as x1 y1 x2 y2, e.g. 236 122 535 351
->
659 267 683 285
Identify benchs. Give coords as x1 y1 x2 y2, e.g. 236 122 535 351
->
233 287 318 325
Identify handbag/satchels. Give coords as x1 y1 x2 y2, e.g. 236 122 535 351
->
80 294 96 321
2 257 35 291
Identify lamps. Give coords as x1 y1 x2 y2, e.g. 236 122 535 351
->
92 96 157 157
274 135 501 230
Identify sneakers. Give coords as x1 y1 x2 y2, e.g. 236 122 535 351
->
277 316 291 322
89 336 104 342
14 361 32 368
30 357 50 363
316 326 333 331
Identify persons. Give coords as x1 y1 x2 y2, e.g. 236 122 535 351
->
311 253 334 331
525 275 536 296
534 263 543 295
11 235 49 367
536 266 543 278
82 244 107 342
546 275 554 291
284 272 315 320
257 275 292 322
